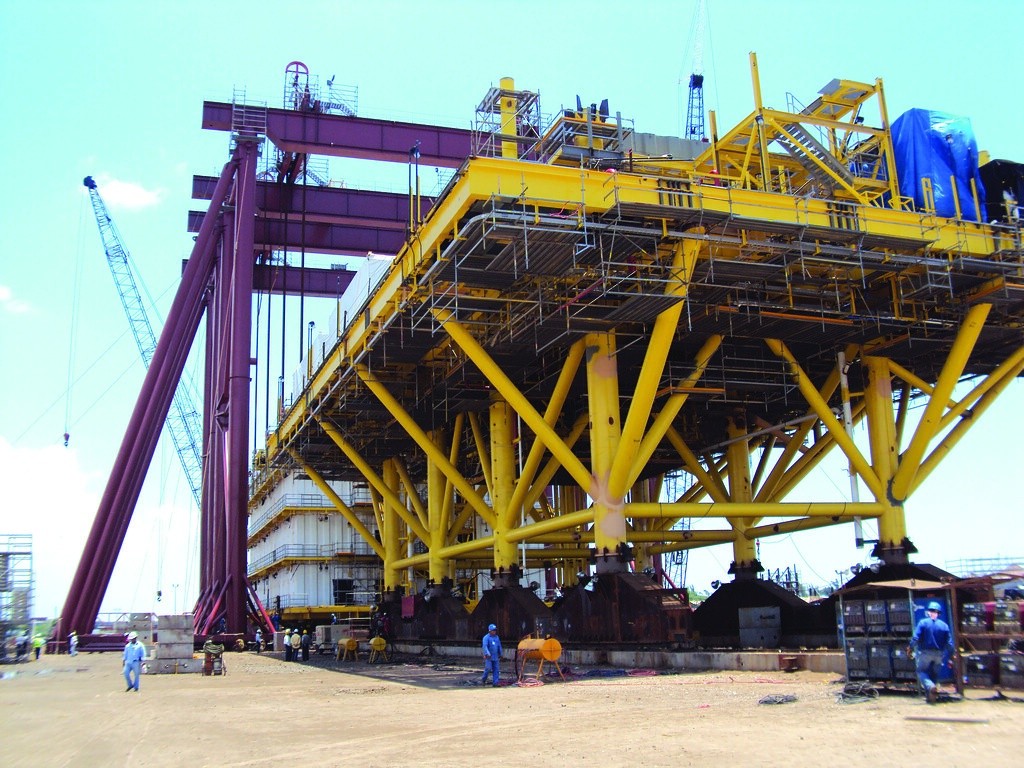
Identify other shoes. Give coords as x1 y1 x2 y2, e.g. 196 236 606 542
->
134 688 138 692
126 686 133 691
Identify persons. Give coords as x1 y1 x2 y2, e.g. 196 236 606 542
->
283 628 309 662
369 606 390 638
479 623 506 689
215 615 225 634
272 613 281 633
329 612 338 626
905 600 957 705
3 631 80 665
119 629 147 692
254 629 263 653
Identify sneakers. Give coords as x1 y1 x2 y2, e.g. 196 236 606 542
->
481 680 486 686
926 683 937 704
493 683 501 687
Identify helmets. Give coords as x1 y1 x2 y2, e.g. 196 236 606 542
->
303 630 308 634
927 601 942 611
285 629 291 634
488 623 497 632
294 628 298 632
331 614 335 616
256 629 261 632
127 632 137 640
